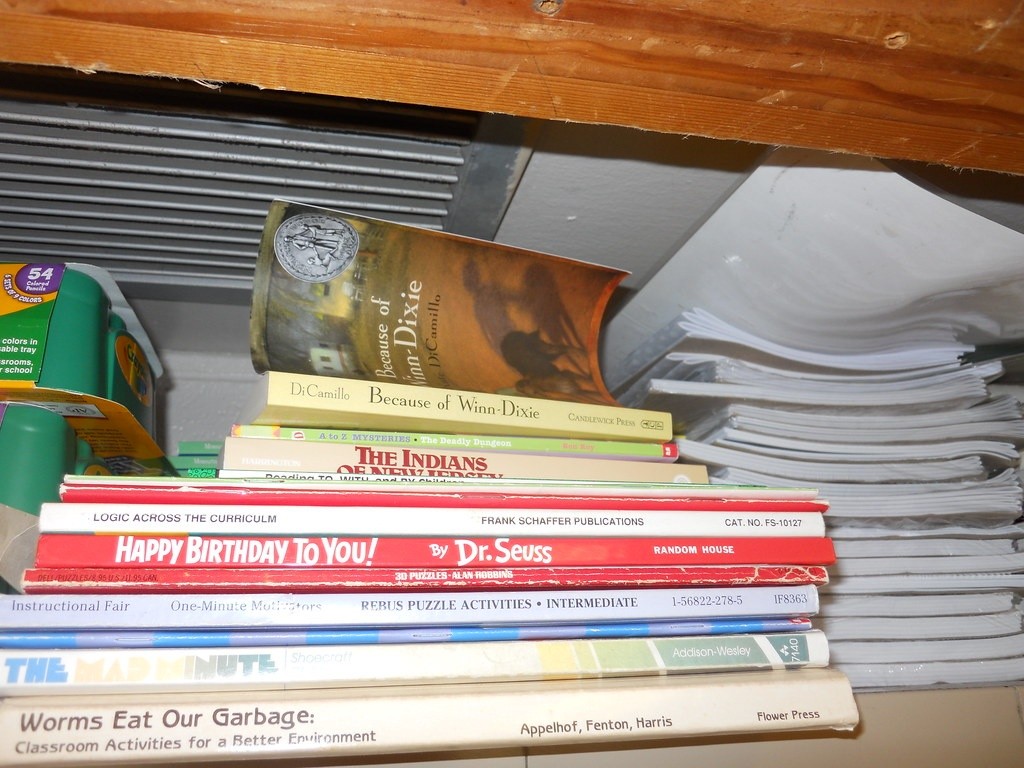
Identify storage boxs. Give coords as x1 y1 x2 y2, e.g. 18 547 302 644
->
0 269 158 595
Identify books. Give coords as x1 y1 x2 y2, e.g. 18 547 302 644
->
0 146 1024 768
